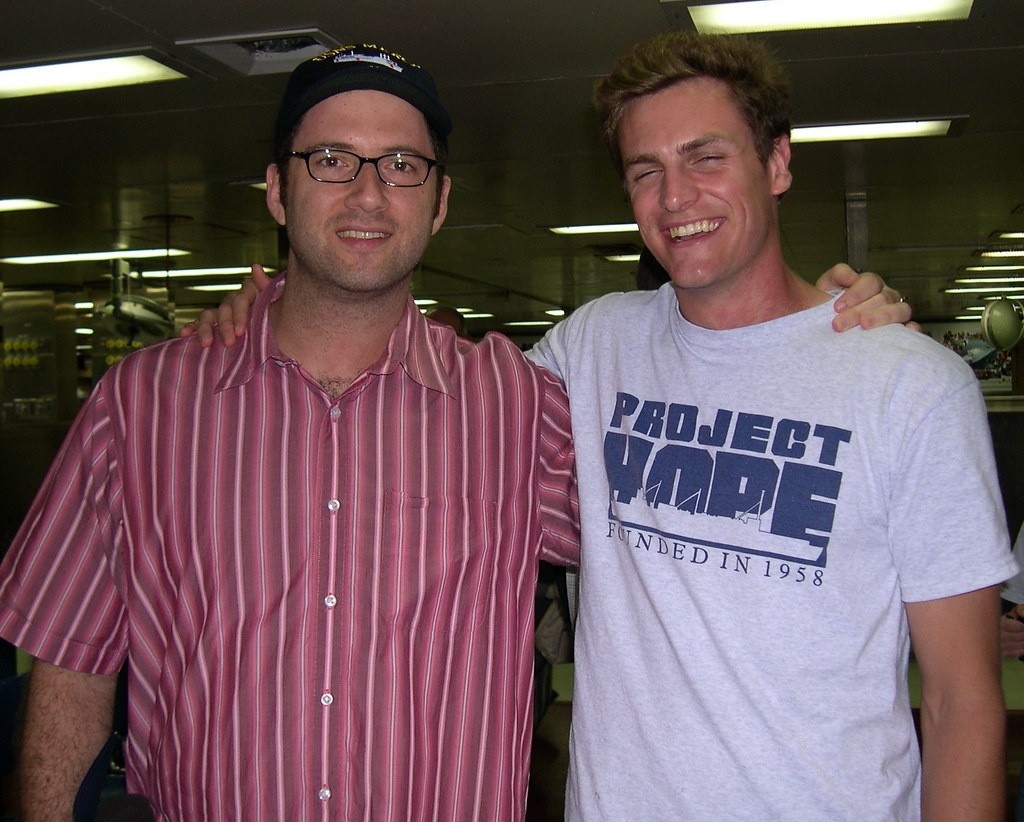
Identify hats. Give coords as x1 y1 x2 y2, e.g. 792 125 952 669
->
279 43 453 164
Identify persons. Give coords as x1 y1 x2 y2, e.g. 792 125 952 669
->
999 522 1024 658
424 304 465 337
0 42 920 822
180 35 1020 822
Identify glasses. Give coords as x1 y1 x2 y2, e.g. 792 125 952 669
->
288 147 439 187
